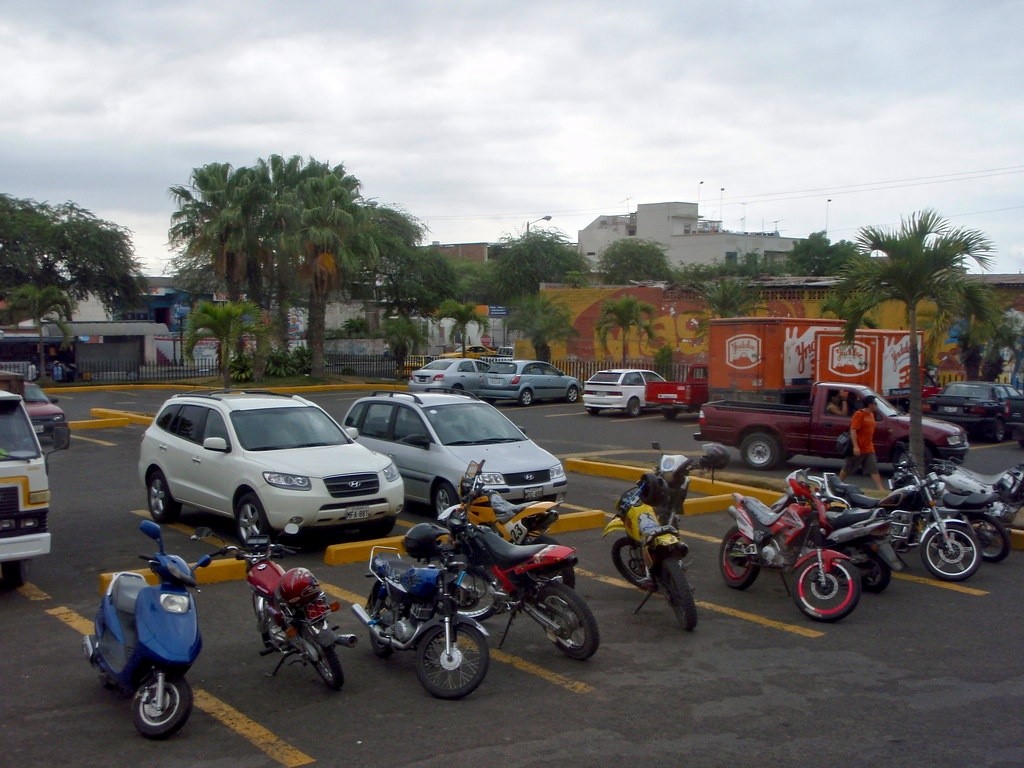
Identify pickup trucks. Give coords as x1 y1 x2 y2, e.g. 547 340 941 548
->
692 382 969 474
1006 396 1024 448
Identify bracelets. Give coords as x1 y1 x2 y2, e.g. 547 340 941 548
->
842 398 847 401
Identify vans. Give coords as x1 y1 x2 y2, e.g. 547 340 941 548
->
0 390 72 588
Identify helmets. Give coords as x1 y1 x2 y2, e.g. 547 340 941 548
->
640 473 669 506
699 442 730 471
278 567 321 607
401 521 451 559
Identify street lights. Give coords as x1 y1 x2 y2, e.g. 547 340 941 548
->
527 215 553 242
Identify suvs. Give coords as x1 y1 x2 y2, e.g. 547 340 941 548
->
342 385 568 524
137 389 405 545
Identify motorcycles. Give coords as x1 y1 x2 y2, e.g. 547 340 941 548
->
603 440 706 632
445 482 601 662
190 522 358 692
770 452 1024 595
351 516 493 700
718 477 862 623
434 458 578 591
80 519 212 741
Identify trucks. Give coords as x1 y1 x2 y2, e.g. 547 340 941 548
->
709 317 847 406
643 363 709 421
813 328 942 402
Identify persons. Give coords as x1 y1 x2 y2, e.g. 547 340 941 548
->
838 396 884 490
53 361 62 381
27 361 39 382
825 390 848 417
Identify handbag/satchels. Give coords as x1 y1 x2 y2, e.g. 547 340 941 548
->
837 434 855 458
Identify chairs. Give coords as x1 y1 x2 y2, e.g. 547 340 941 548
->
438 408 466 442
403 412 424 437
369 416 387 432
632 377 644 387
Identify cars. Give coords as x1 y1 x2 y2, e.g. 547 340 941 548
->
479 359 584 407
407 358 497 402
498 345 515 355
438 345 499 360
922 381 1023 443
584 369 670 417
21 381 66 438
395 354 432 379
